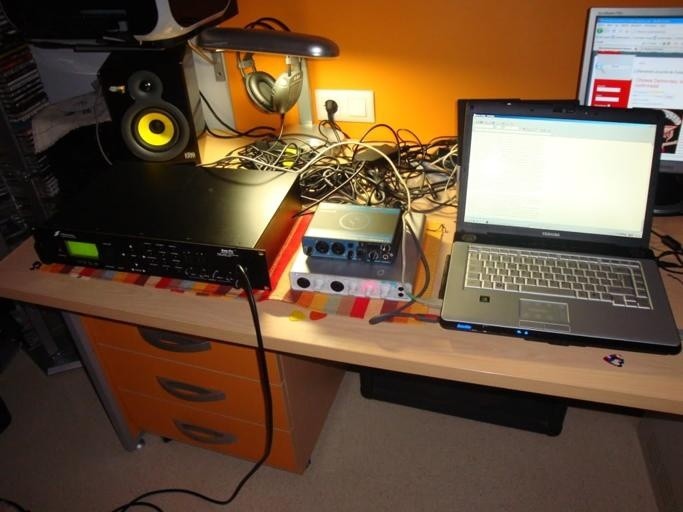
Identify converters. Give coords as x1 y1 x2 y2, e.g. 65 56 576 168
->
354 144 399 165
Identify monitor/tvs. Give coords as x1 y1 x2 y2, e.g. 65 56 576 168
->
575 7 683 215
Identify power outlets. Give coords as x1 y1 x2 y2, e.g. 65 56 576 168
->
314 88 344 123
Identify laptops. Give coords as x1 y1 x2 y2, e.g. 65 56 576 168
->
440 101 681 358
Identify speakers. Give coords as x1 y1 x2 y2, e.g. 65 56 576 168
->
97 41 209 169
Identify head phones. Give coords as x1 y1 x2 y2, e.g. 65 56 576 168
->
235 16 305 117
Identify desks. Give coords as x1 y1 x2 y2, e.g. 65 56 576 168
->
3 125 681 475
